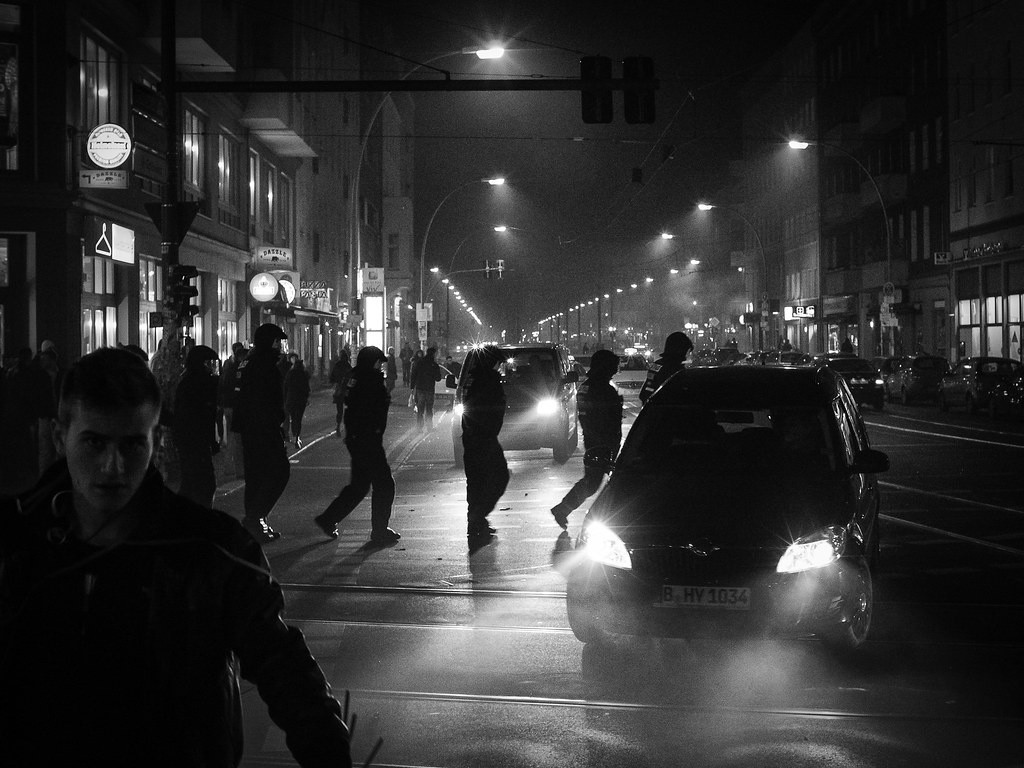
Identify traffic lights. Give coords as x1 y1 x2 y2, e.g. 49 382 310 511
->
175 265 198 327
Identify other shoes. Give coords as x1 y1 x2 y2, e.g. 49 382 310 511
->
296 438 302 449
314 515 339 539
466 524 496 543
371 527 400 540
240 515 281 541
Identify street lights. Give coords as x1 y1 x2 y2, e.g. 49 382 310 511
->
537 260 705 359
699 206 768 353
662 233 721 348
351 41 513 350
416 175 507 361
789 136 895 356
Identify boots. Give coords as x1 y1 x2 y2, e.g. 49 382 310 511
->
550 501 573 530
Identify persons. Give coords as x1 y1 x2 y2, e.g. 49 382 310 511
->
0 322 455 543
0 347 353 768
461 347 508 550
551 351 624 531
526 354 553 382
842 339 854 353
725 336 738 347
779 339 792 352
639 331 694 407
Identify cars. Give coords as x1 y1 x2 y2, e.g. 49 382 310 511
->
689 347 816 367
812 354 883 412
939 356 1024 422
884 353 947 406
614 355 650 399
567 366 890 653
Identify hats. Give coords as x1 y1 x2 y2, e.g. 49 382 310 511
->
254 323 289 339
356 346 389 364
186 345 219 364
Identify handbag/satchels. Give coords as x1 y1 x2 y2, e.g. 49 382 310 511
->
408 394 415 407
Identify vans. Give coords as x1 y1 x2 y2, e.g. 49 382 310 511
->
450 342 580 464
